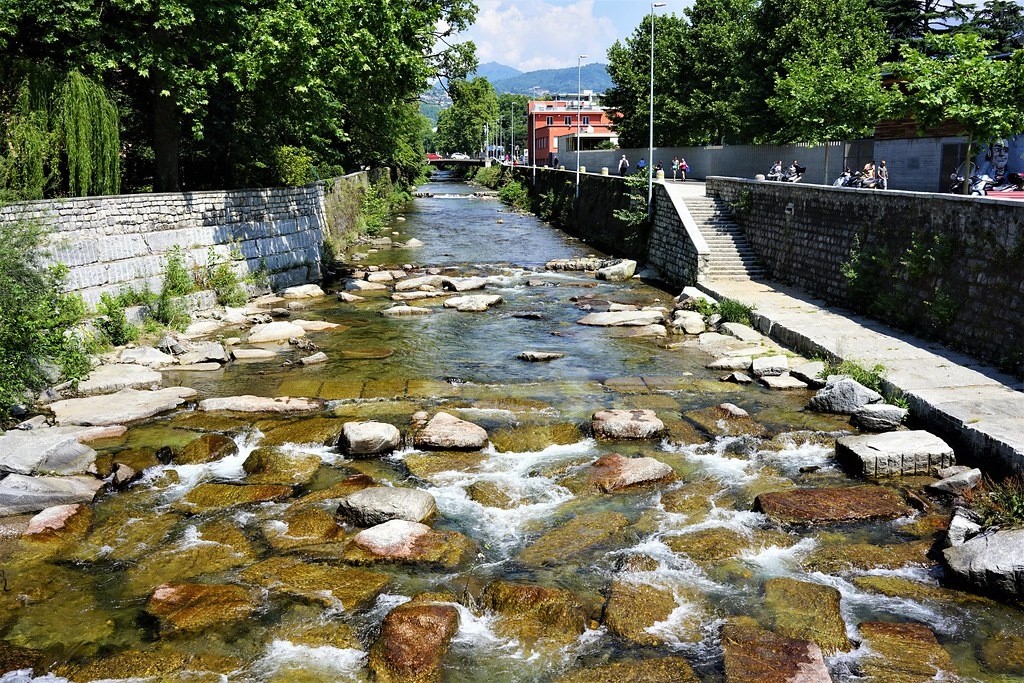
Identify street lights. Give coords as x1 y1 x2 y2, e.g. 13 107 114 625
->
511 102 517 174
576 54 590 198
646 2 667 223
500 118 506 164
532 85 541 186
514 120 524 156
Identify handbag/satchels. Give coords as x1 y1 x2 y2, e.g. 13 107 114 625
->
672 166 677 170
686 166 690 172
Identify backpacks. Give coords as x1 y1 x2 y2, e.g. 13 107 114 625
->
621 160 627 169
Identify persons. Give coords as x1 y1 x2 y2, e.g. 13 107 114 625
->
770 160 802 182
678 158 688 181
553 157 559 169
654 161 663 172
636 157 647 179
501 154 509 163
671 156 679 182
618 155 629 177
864 160 888 190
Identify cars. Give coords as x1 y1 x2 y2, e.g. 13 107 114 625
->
425 152 443 159
450 152 470 161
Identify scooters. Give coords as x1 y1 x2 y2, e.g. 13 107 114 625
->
949 160 1024 197
781 165 806 183
832 165 885 190
